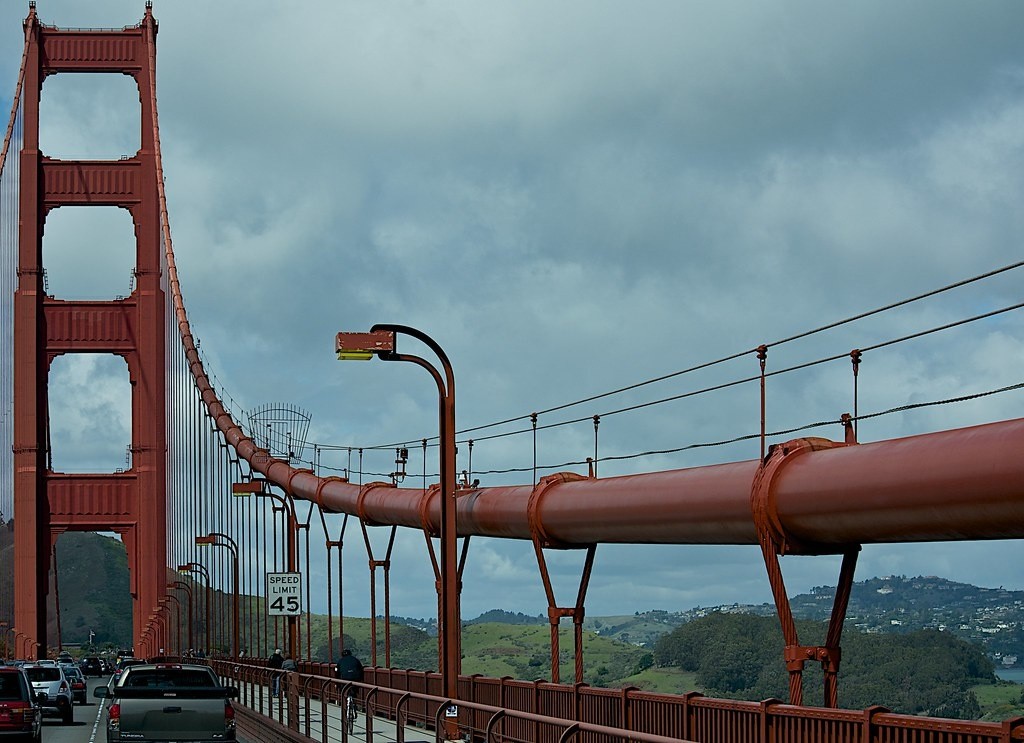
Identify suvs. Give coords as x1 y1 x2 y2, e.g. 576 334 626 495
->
19 663 74 725
0 666 49 743
80 658 102 677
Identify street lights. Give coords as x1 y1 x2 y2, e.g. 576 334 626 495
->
167 582 192 655
194 534 239 659
334 323 460 740
178 564 211 659
231 483 296 667
136 595 181 658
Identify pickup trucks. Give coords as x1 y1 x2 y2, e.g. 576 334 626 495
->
57 658 74 667
92 664 239 743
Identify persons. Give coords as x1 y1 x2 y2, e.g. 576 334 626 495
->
268 649 284 698
177 649 205 665
335 649 364 719
281 653 298 700
239 650 247 658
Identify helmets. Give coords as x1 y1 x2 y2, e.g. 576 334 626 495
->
342 649 351 656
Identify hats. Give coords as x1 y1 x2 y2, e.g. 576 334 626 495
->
275 649 282 653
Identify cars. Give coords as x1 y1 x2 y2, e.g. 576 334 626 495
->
4 660 56 667
99 658 112 673
113 661 148 688
60 666 90 704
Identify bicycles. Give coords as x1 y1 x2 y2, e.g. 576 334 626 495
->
344 693 354 734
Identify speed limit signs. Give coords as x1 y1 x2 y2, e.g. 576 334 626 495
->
266 572 301 615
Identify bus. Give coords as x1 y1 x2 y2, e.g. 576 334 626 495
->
116 649 133 666
